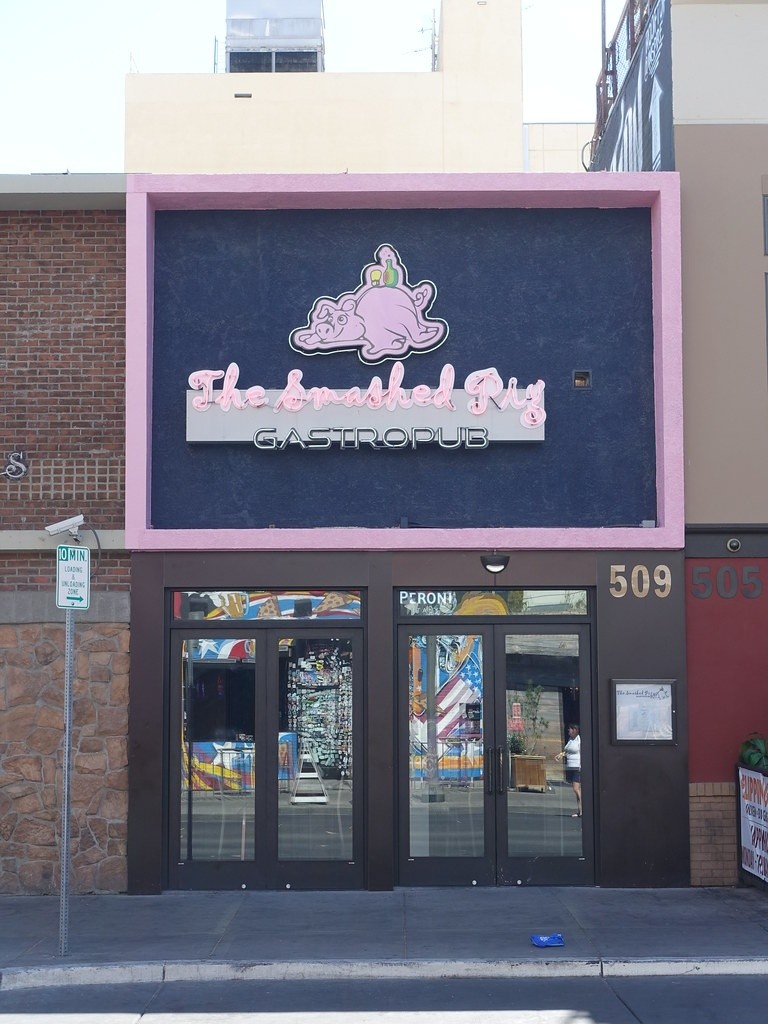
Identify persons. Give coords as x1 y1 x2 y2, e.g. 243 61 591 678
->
553 724 583 817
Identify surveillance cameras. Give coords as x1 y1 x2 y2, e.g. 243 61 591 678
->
45 514 84 536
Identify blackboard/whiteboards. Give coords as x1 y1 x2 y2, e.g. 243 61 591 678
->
290 737 328 803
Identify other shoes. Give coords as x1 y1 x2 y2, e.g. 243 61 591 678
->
572 812 582 817
